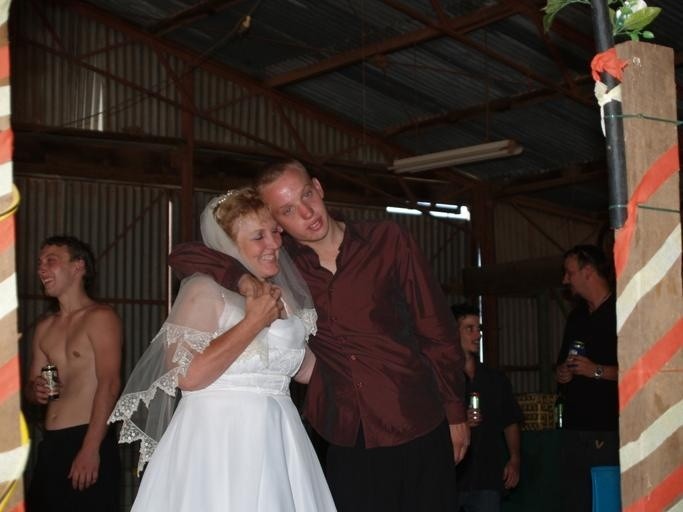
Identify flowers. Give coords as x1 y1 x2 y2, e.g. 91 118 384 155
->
538 0 663 43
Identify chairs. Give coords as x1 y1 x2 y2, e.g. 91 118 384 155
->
590 465 622 512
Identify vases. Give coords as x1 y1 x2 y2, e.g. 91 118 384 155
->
512 389 561 434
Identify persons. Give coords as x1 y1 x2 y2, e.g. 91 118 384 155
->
24 234 125 512
543 244 619 511
167 156 472 512
105 187 339 512
450 303 525 512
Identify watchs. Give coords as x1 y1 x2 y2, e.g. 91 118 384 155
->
594 364 604 380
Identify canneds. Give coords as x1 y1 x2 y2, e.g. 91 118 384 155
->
465 392 481 427
565 340 585 368
40 365 60 400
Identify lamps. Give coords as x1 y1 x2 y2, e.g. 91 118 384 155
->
384 26 523 173
